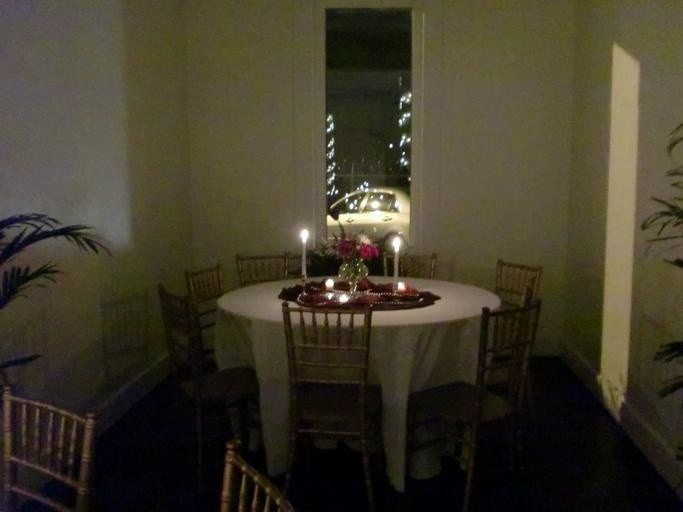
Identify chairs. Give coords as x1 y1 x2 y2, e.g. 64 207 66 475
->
184 260 228 374
381 253 442 279
280 298 386 512
217 435 296 512
234 251 290 289
156 282 259 496
484 259 544 433
0 387 95 512
404 298 543 511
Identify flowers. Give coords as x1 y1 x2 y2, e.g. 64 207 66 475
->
314 221 381 281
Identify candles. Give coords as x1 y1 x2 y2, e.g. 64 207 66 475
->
301 242 307 276
394 250 398 287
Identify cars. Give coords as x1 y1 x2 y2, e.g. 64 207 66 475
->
327 186 409 243
